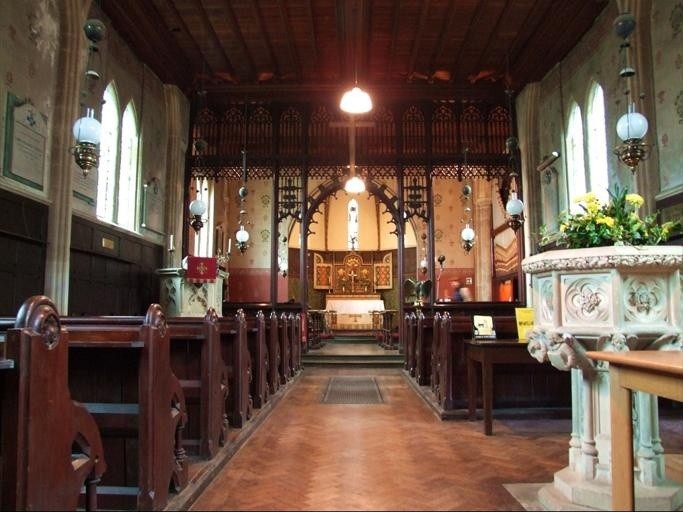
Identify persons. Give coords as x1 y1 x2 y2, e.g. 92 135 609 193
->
447 277 472 302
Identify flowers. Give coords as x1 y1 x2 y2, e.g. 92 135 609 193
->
527 180 681 250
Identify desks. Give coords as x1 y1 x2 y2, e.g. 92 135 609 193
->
462 338 551 434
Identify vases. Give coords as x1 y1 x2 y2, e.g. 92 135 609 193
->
519 244 682 511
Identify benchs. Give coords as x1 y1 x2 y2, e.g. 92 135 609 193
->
0 295 301 512
402 302 571 422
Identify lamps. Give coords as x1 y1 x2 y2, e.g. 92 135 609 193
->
66 1 106 179
188 88 208 234
458 58 475 253
504 33 524 234
234 92 250 255
613 1 654 178
